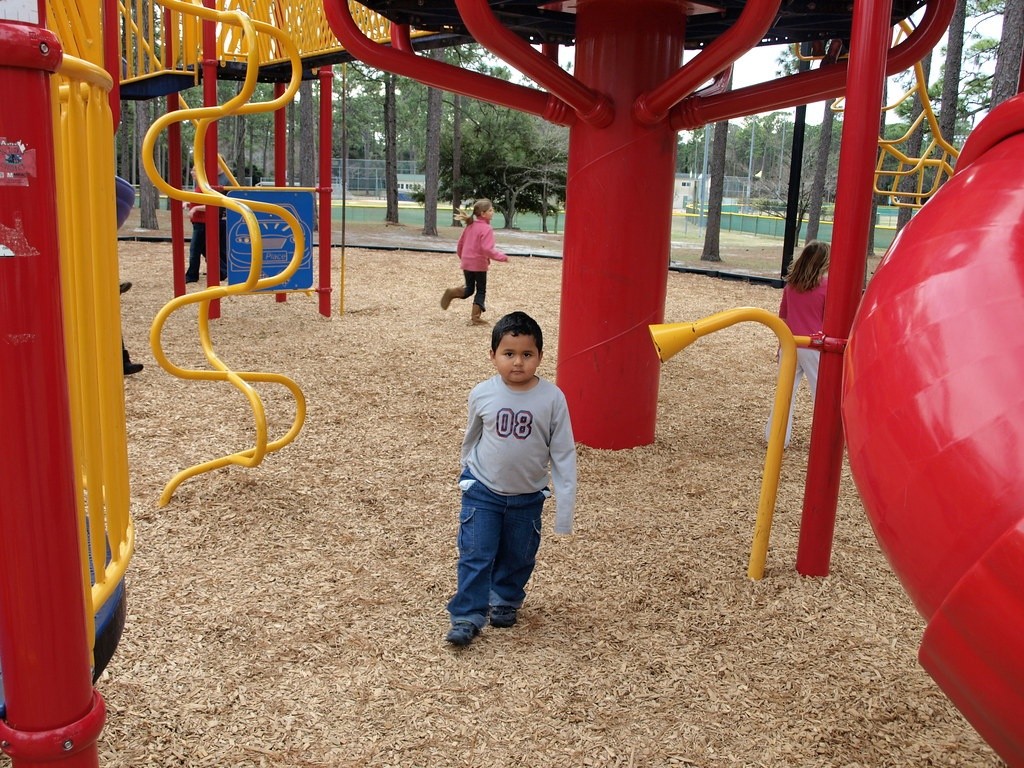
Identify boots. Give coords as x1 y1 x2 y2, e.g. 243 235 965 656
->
123 349 143 375
440 285 466 310
471 303 486 325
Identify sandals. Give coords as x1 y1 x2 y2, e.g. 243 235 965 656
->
446 621 479 646
489 604 517 628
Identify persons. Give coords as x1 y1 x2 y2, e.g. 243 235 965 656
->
765 240 830 447
442 311 578 642
440 199 510 324
182 155 234 284
120 283 144 375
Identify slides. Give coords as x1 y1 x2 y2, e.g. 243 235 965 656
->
838 92 1024 768
115 175 135 230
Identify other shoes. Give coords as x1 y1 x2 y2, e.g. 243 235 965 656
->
185 274 197 283
119 282 132 294
